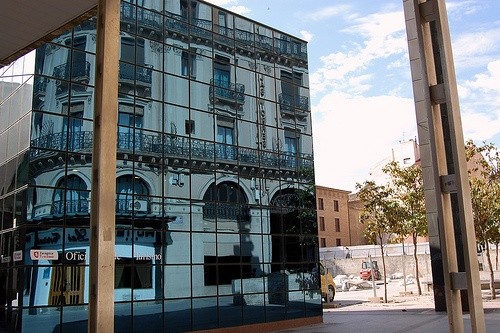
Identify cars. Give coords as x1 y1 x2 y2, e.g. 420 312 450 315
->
361 261 381 281
319 263 336 303
